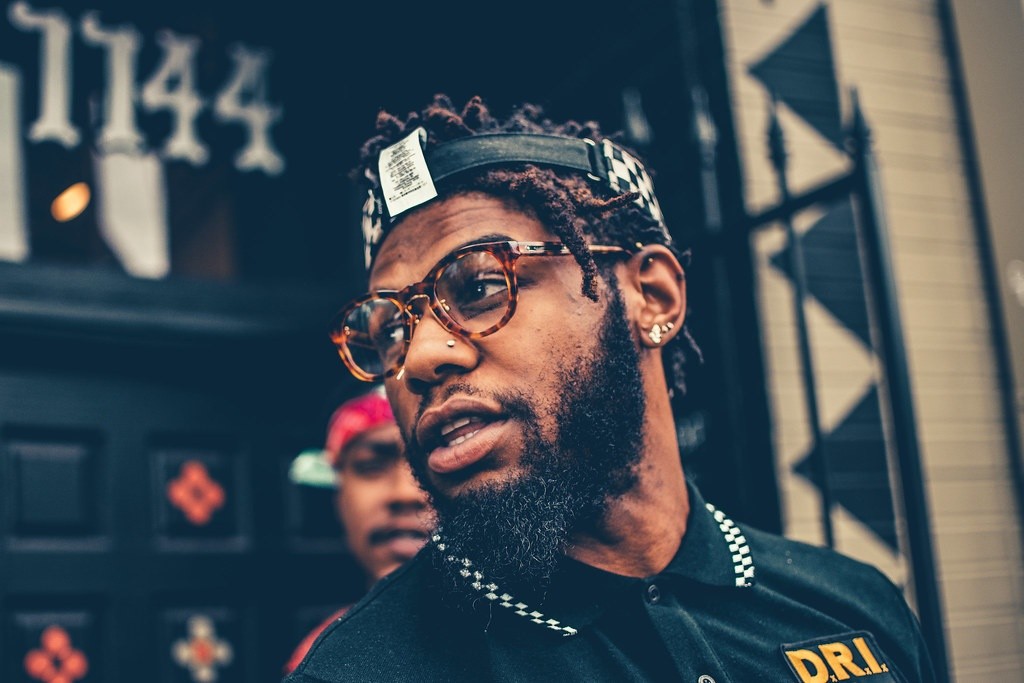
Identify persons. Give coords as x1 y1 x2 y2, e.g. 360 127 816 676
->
284 361 441 673
275 94 937 683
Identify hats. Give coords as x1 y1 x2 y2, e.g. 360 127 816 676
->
326 383 399 467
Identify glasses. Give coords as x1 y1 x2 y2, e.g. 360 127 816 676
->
325 241 634 381
